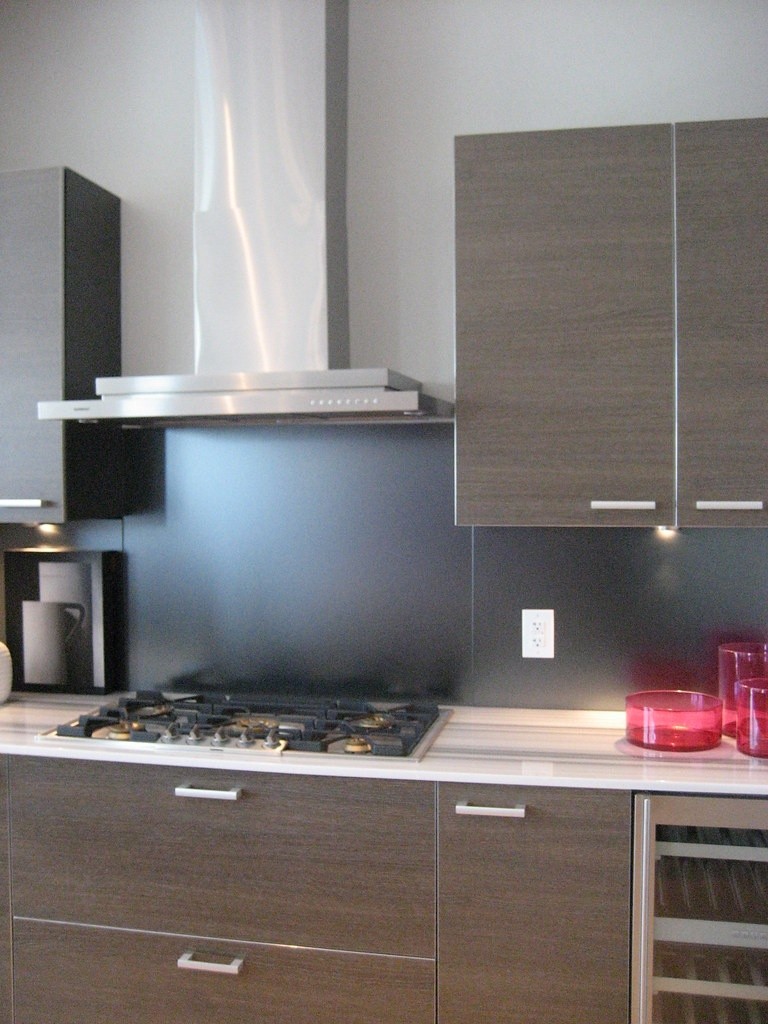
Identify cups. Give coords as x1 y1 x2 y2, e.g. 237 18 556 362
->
718 642 768 738
23 600 85 686
733 678 768 759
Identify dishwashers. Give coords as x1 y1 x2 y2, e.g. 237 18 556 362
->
632 791 768 1024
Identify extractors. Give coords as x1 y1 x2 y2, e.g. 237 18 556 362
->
35 0 455 430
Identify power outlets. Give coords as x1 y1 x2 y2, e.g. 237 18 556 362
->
522 608 555 659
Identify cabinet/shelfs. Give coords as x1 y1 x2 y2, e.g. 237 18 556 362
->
1 164 124 526
453 117 768 530
0 753 439 1024
435 782 633 1024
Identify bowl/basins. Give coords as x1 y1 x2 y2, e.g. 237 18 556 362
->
624 689 723 753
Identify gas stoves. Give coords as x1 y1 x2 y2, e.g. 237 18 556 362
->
34 690 455 764
